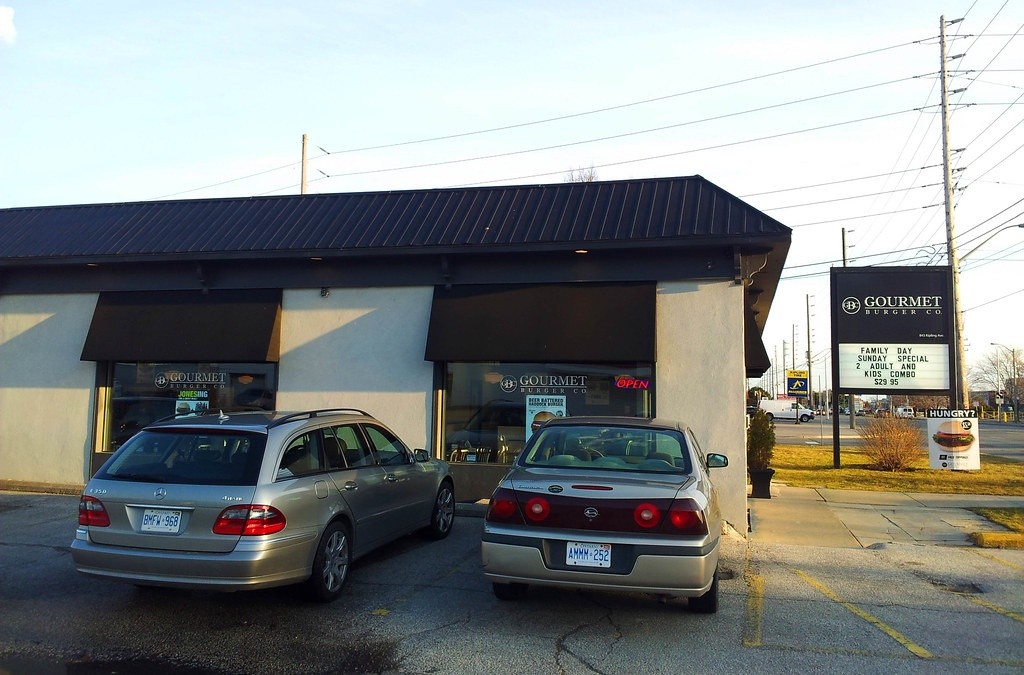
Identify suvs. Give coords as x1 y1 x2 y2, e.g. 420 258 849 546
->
446 398 528 460
70 408 455 606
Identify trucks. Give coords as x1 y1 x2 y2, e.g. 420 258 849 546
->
760 400 815 423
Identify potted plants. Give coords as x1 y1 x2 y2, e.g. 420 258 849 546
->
747 407 779 498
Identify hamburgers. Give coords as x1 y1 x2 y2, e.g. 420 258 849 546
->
177 402 190 414
933 421 975 452
531 411 556 433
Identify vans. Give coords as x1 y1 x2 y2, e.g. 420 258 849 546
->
895 409 915 420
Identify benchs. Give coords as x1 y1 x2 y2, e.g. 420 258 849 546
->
547 454 684 472
175 450 242 482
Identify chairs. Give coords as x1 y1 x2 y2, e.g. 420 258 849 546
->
563 449 592 462
644 452 675 466
231 451 246 465
324 436 347 469
499 436 521 464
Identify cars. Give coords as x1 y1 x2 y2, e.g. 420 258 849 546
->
480 416 730 618
828 408 833 415
874 409 887 418
856 409 866 417
816 409 825 415
845 410 850 415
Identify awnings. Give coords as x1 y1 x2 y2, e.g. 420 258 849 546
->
422 282 656 366
80 288 281 365
743 309 771 378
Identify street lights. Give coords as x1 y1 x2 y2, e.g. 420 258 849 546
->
948 221 1024 411
990 342 1019 422
